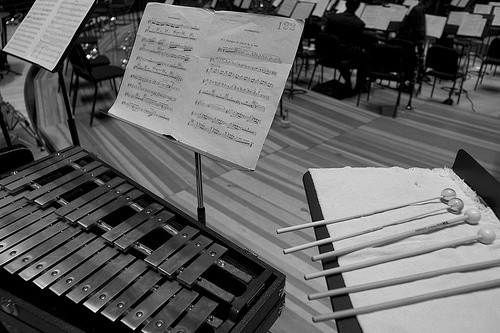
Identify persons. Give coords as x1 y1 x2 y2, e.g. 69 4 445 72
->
314 0 431 82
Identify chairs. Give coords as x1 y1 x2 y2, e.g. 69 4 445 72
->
298 22 500 119
64 0 132 127
